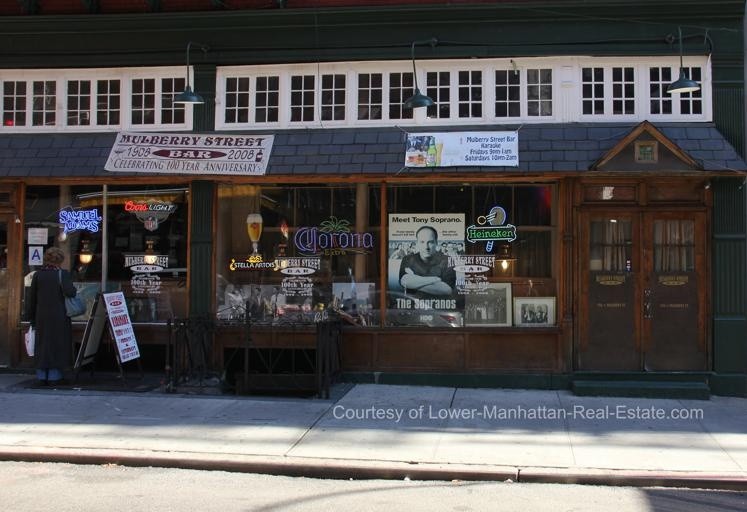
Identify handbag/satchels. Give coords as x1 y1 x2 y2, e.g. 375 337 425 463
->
25 326 35 357
63 291 85 317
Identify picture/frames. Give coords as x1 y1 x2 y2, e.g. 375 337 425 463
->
463 280 513 328
512 296 556 328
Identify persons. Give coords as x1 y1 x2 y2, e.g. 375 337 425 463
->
390 243 464 259
249 288 267 314
269 286 287 308
536 305 546 322
26 247 76 387
398 226 457 297
523 306 536 322
228 289 243 306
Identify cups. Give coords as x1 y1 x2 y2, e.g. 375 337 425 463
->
434 137 445 166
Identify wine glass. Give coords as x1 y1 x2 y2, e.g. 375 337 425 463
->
245 214 263 257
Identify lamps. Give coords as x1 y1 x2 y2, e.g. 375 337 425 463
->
172 40 208 105
144 238 158 265
76 239 95 263
403 39 435 109
665 26 701 94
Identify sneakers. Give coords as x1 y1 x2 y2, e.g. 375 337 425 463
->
34 380 67 386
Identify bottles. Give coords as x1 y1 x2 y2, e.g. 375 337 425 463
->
425 136 438 167
